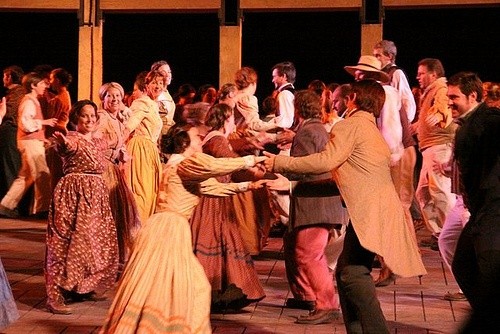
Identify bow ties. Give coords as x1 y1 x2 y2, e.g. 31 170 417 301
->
454 118 466 126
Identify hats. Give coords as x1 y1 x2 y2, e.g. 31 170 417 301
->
344 55 391 83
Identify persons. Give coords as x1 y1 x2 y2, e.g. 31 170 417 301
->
433 83 500 299
447 72 500 324
151 61 176 135
260 63 295 234
92 82 141 274
280 90 345 325
46 69 71 185
233 67 270 135
195 104 267 304
32 65 56 118
373 42 426 231
99 126 271 334
133 74 355 137
263 81 426 334
344 56 417 287
0 66 28 216
44 100 120 314
1 75 52 218
409 59 453 250
124 71 166 227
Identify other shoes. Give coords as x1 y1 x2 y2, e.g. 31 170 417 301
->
374 266 396 286
32 211 49 219
297 310 341 324
420 235 439 251
47 301 72 315
286 298 315 310
91 293 108 300
0 205 21 218
443 292 468 301
